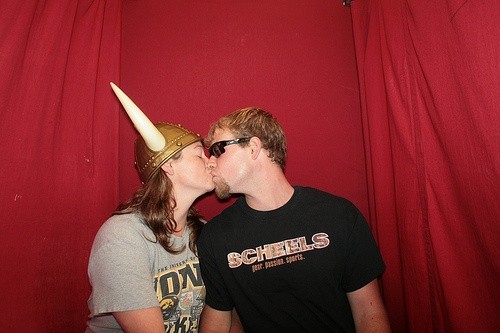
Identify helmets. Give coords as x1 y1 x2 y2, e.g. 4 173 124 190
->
136 121 205 184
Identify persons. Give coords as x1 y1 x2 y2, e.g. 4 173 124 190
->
85 80 242 333
199 109 394 333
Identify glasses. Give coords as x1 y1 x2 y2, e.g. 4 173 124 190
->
209 136 252 158
